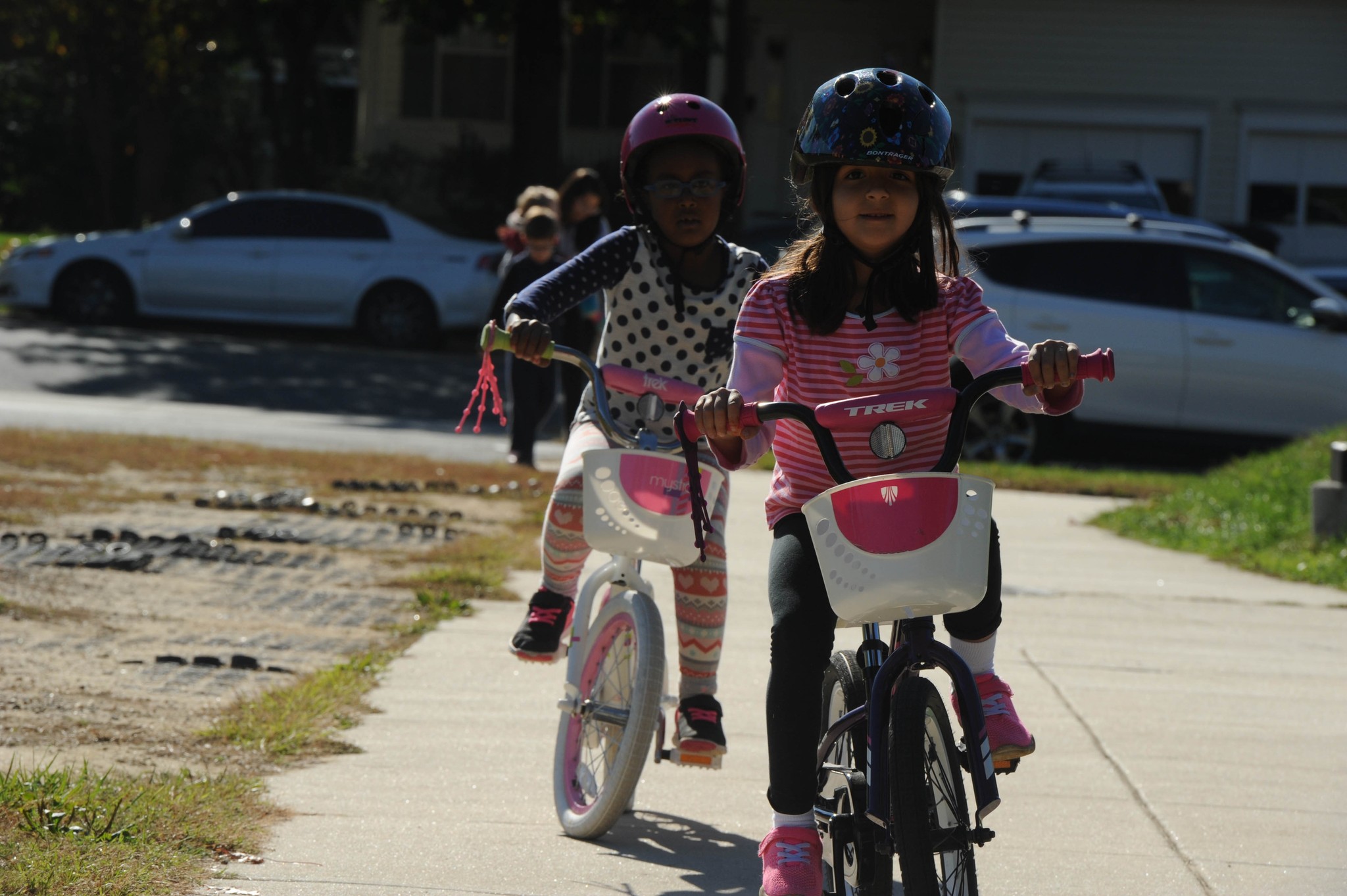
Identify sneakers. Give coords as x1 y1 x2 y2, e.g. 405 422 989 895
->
509 587 576 662
950 674 1036 762
672 693 726 753
759 825 824 895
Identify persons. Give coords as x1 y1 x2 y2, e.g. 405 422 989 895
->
503 91 773 755
488 167 615 465
693 68 1087 896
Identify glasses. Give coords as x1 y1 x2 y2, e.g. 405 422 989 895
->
644 176 722 200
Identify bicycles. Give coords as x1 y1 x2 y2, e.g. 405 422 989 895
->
481 320 732 836
674 346 1120 880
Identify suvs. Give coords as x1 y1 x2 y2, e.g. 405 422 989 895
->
939 213 1347 460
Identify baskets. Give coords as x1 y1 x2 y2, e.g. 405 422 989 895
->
799 470 995 623
581 450 726 569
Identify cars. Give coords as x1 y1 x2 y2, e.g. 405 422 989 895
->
1020 159 1175 220
8 188 505 350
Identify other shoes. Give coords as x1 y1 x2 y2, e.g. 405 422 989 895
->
510 451 533 470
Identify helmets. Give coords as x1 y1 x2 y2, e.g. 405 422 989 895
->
619 93 743 215
792 68 953 179
